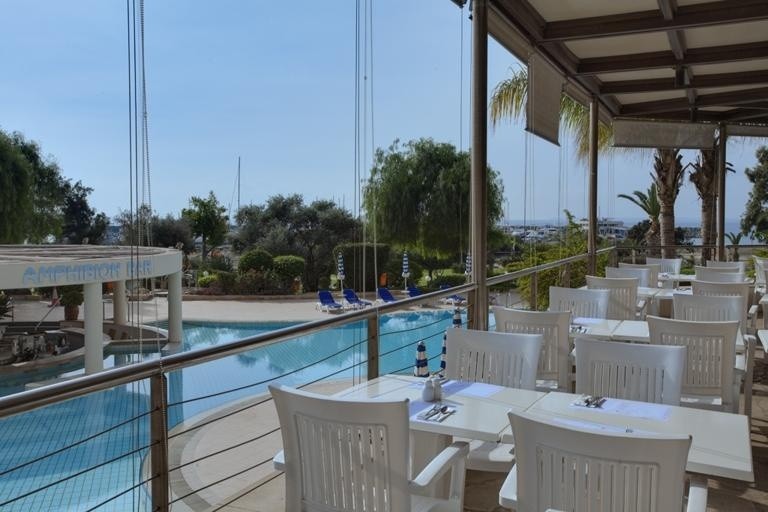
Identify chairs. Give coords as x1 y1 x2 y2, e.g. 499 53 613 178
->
644 312 736 413
265 379 471 512
440 325 544 475
571 334 689 409
540 252 767 353
488 305 574 393
496 406 712 512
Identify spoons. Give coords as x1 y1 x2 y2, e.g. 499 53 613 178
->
425 405 450 421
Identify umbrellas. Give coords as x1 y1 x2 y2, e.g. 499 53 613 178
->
402 250 410 296
465 250 472 275
337 251 346 314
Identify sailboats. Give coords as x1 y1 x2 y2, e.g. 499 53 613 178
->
194 157 243 252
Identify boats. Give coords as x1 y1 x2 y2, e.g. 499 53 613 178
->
505 217 632 246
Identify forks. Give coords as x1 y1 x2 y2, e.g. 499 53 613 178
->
584 395 604 409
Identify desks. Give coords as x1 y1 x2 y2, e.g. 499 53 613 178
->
311 373 548 510
499 390 759 489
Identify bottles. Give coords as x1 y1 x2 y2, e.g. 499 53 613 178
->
422 378 442 402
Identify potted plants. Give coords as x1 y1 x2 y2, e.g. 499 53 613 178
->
58 289 84 321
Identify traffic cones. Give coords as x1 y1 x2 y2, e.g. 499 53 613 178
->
47 286 63 308
